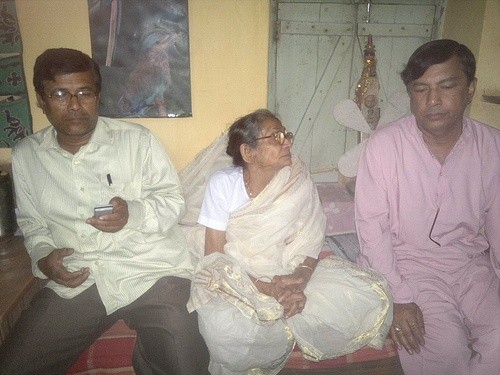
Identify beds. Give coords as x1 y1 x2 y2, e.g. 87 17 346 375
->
0 177 404 375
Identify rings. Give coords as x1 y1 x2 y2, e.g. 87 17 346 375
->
395 328 401 331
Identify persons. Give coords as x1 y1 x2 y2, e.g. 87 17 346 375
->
186 109 393 375
355 39 500 375
0 48 210 375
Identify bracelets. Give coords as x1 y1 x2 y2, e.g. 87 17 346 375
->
295 266 313 271
255 276 272 286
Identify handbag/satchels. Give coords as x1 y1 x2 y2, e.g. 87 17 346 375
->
206 257 284 327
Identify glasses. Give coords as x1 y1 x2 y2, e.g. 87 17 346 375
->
255 132 294 145
44 87 97 106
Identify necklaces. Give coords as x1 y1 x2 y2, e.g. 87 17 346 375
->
243 171 253 198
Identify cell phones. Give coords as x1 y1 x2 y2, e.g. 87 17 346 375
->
94 204 114 218
